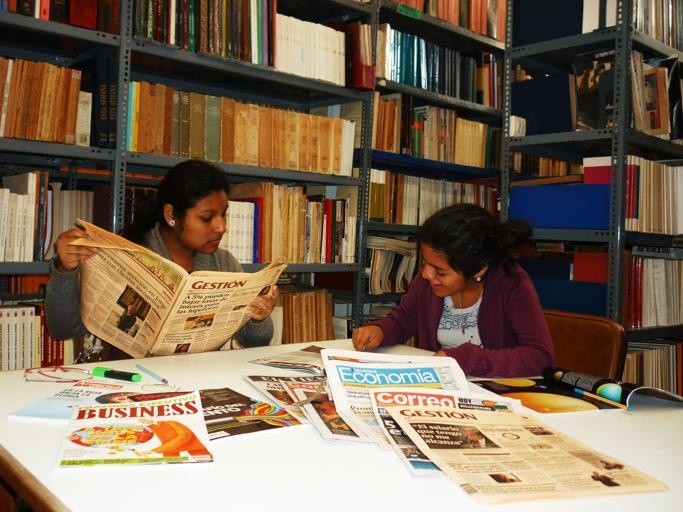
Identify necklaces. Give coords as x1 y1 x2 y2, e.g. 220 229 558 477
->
457 287 483 335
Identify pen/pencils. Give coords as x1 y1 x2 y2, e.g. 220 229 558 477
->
136 363 168 384
574 387 632 411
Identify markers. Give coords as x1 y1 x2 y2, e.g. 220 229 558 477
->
92 367 141 382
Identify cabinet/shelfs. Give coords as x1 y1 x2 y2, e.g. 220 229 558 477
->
0 0 126 370
116 0 377 369
361 0 513 338
501 1 681 397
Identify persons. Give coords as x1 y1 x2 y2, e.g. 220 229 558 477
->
461 426 493 449
351 204 557 379
185 314 213 330
591 470 622 486
491 474 519 483
116 305 137 333
95 391 140 404
174 343 190 353
600 460 624 470
46 158 279 364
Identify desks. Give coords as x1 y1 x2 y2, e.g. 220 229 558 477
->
1 336 682 512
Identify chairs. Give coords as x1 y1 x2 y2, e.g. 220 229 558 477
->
536 308 627 383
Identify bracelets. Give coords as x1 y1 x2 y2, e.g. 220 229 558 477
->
59 260 80 271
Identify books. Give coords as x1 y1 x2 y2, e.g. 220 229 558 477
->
582 0 683 52
583 156 683 241
0 57 118 150
125 81 355 179
509 175 583 188
352 168 500 226
376 21 505 110
365 233 417 294
468 364 683 417
46 332 104 367
57 389 214 469
0 0 122 35
309 90 501 171
131 1 276 70
47 181 157 262
1 273 46 372
509 153 583 175
506 240 683 331
218 274 351 351
389 0 506 44
577 52 673 141
274 10 376 91
509 64 577 136
218 182 358 263
622 337 682 395
351 295 398 326
1 170 46 263
